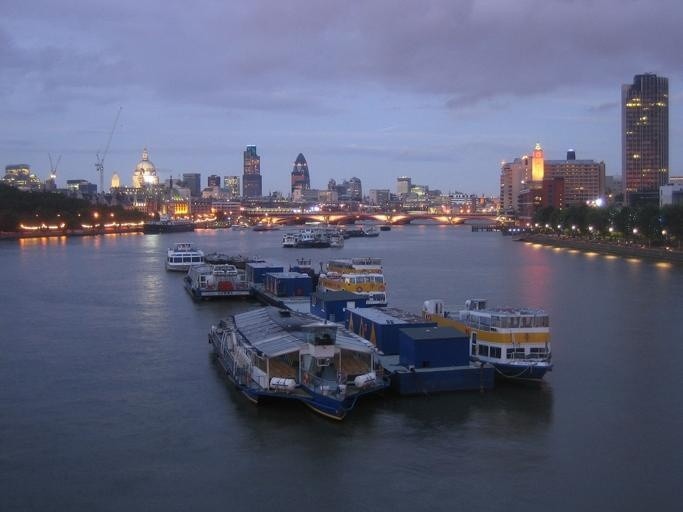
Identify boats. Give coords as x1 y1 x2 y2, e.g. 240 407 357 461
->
252 222 279 231
142 213 196 234
228 224 242 231
163 241 205 272
280 225 380 249
378 224 390 231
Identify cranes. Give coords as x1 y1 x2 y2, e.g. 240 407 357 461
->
47 150 64 187
93 104 123 193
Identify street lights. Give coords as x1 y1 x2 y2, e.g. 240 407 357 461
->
660 229 668 249
632 228 639 248
525 223 593 242
607 225 617 245
